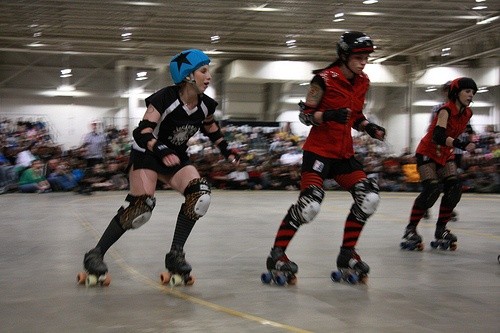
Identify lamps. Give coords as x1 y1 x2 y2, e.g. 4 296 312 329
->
332 3 346 23
474 13 492 26
470 0 488 10
135 69 149 81
119 29 133 42
440 45 453 57
425 86 438 92
56 54 76 91
285 38 297 48
211 34 220 44
476 87 488 93
33 29 43 39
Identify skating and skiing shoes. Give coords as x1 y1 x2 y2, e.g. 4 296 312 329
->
261 250 297 286
430 225 458 250
331 248 369 284
401 228 424 252
77 247 110 288
160 253 194 288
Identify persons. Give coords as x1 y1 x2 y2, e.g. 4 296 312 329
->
81 49 239 280
400 77 481 243
265 31 388 274
0 113 500 195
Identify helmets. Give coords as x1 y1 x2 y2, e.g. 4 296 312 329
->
169 49 210 83
337 30 374 56
449 76 478 97
